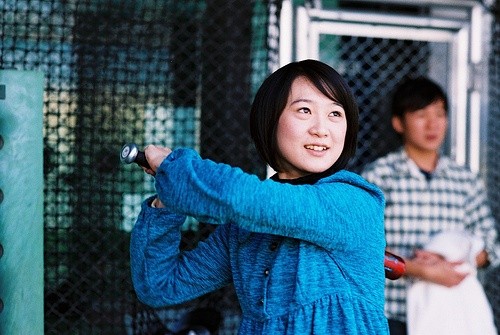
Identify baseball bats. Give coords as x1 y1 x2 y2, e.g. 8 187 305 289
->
120 143 406 282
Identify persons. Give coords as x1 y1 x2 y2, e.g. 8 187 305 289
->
354 68 500 335
127 60 392 335
